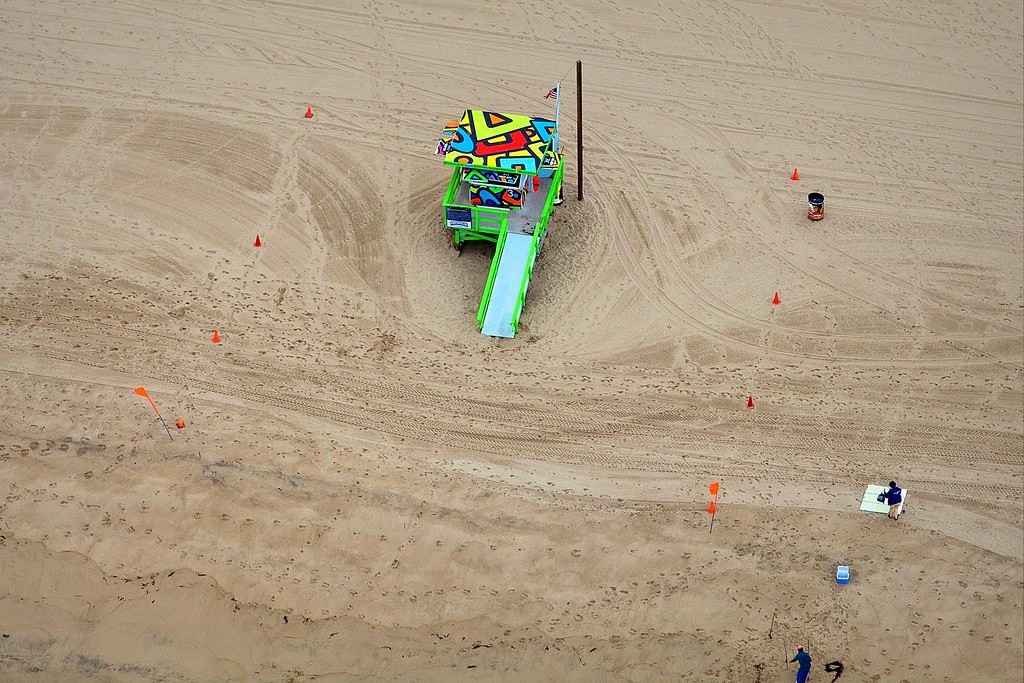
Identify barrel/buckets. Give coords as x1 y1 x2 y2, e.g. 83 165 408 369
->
808 193 825 221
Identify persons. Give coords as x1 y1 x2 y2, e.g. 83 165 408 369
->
789 645 811 683
884 481 901 521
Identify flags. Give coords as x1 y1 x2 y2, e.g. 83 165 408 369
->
709 482 719 496
133 387 157 414
544 87 558 100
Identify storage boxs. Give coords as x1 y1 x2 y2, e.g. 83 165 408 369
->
836 565 850 584
